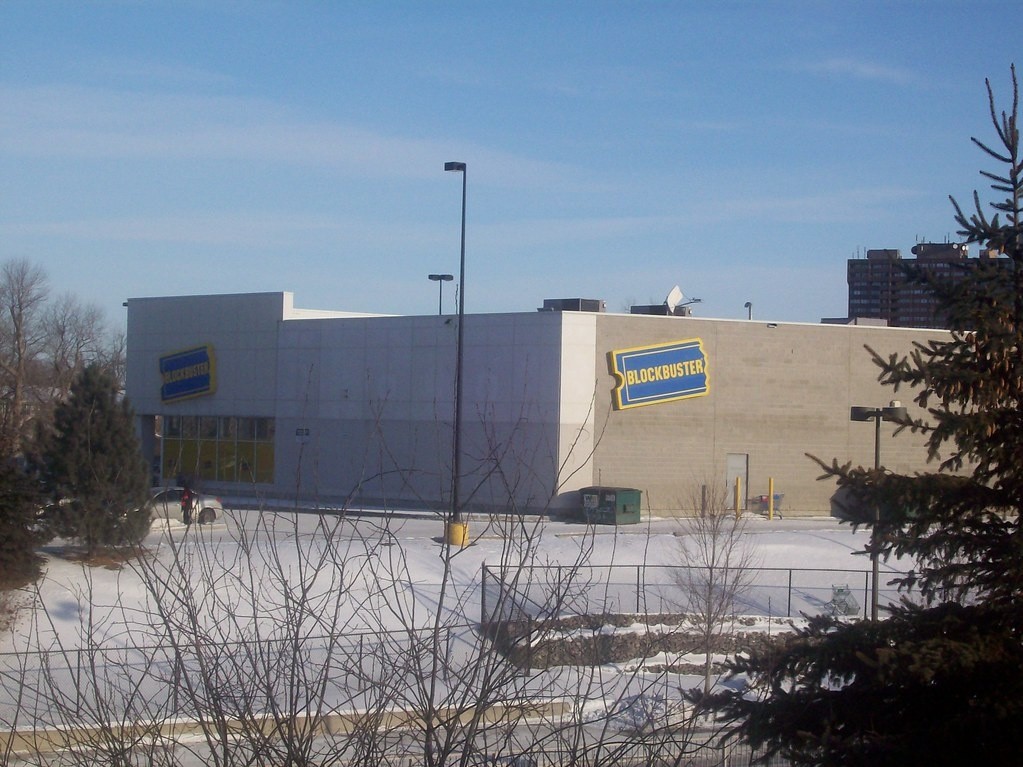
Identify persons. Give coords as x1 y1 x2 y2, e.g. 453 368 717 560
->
180 483 193 525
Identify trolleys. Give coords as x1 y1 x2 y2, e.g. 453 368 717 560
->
758 493 784 520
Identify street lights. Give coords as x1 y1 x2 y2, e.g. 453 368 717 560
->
428 275 453 315
444 161 467 523
850 407 907 619
745 302 752 320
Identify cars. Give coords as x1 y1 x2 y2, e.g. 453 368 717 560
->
120 487 224 524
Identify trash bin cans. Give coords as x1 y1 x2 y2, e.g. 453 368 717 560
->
579 486 643 526
